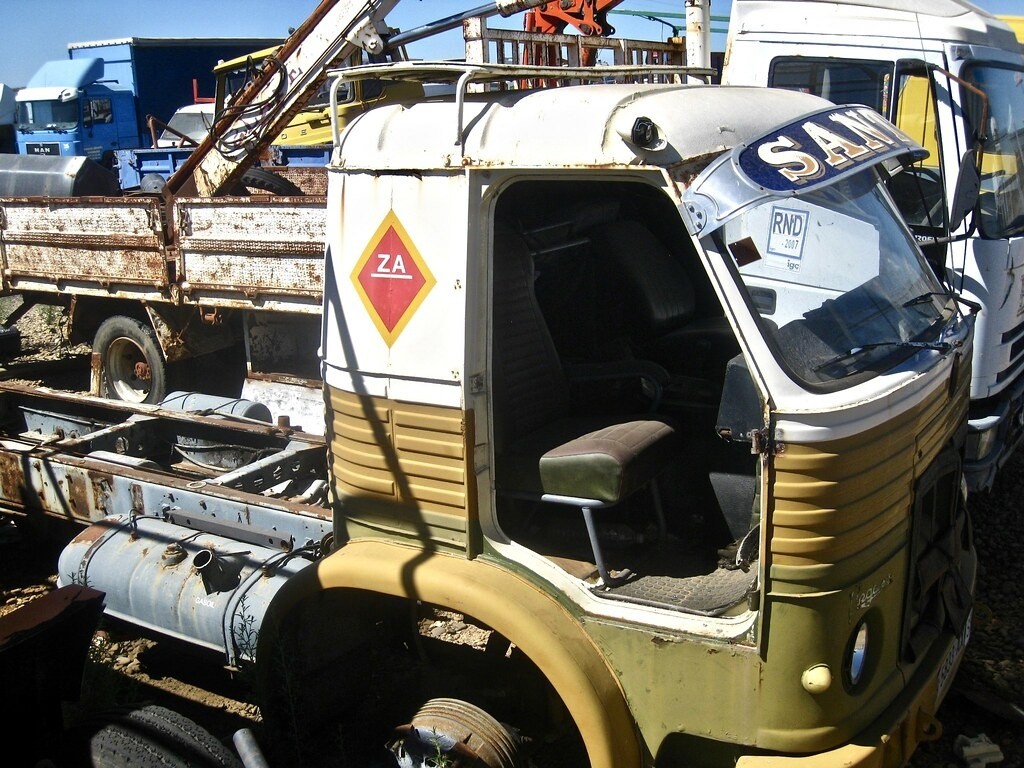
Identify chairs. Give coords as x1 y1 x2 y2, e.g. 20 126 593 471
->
594 221 778 358
493 223 676 592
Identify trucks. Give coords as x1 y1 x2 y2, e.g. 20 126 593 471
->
0 0 1024 768
